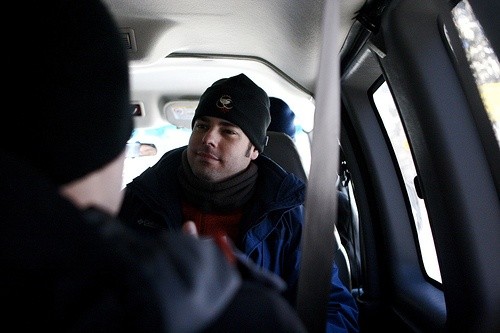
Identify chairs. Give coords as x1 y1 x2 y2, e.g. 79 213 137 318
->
261 132 353 294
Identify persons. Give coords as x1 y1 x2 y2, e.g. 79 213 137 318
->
112 73 362 333
0 0 310 333
265 97 351 254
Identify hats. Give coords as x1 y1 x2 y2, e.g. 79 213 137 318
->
191 74 272 156
0 0 134 186
266 97 295 141
